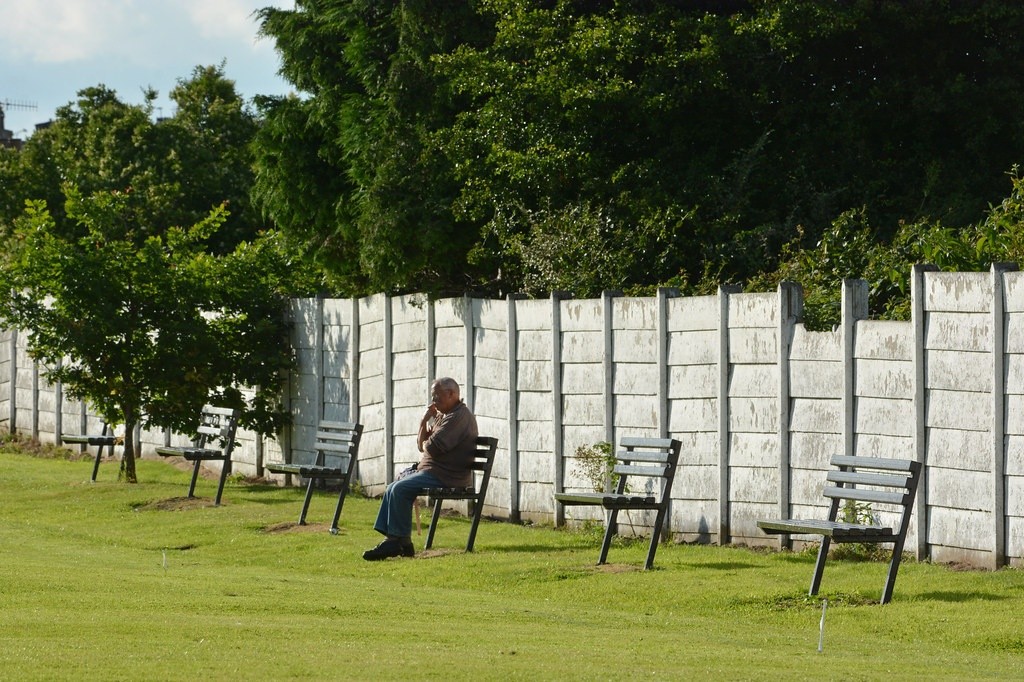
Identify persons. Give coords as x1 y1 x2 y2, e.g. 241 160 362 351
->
361 378 477 563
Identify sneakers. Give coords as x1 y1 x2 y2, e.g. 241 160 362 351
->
399 542 415 558
363 540 403 561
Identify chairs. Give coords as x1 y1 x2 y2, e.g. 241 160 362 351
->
552 436 683 572
154 402 242 506
263 418 365 537
415 435 499 555
59 418 135 484
756 452 924 608
